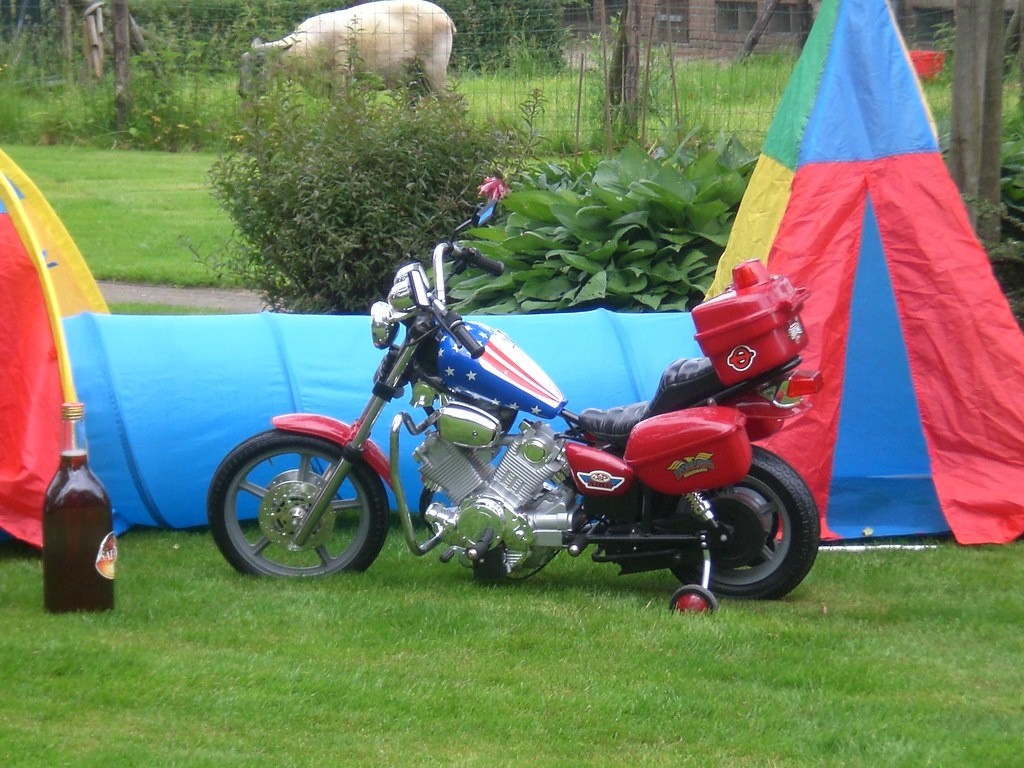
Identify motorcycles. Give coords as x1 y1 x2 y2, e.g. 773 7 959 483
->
210 200 824 621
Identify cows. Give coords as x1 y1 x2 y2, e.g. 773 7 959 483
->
237 0 457 99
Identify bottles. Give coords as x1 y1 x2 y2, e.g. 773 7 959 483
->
42 403 116 614
